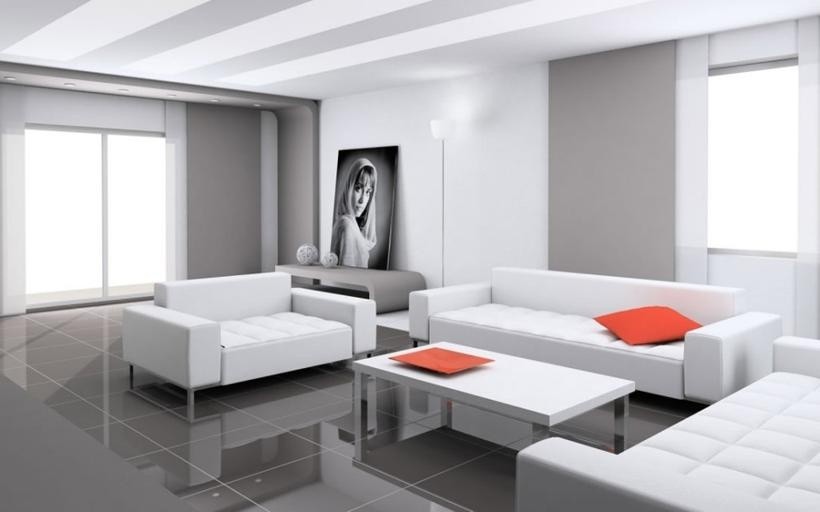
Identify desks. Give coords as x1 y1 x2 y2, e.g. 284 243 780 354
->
271 261 427 312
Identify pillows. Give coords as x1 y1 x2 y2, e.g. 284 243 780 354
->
593 305 704 346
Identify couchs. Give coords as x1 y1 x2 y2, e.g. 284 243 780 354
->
514 336 818 512
123 371 351 486
123 272 379 403
407 265 784 405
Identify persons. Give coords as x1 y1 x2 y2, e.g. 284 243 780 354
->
330 157 378 268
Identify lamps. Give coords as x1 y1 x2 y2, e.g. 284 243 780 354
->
429 119 454 287
